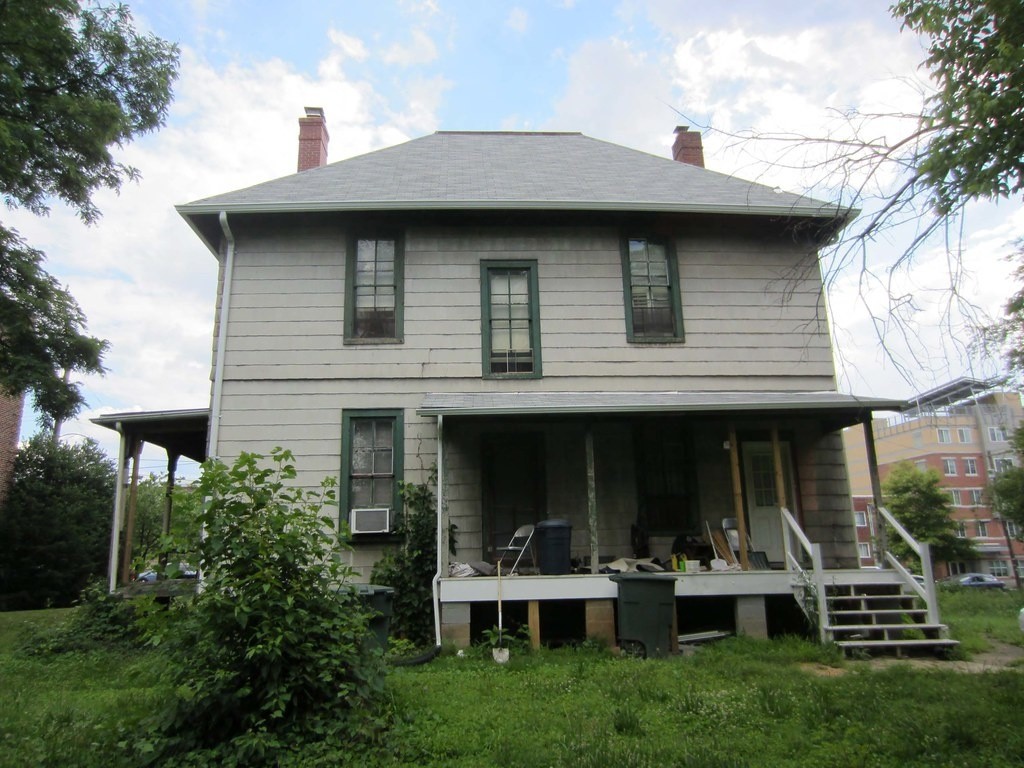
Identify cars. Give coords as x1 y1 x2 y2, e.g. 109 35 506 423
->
911 575 939 588
935 571 1006 589
135 561 197 579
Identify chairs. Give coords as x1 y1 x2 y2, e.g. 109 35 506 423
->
492 525 534 576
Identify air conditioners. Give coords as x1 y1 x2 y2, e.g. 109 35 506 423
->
349 508 389 534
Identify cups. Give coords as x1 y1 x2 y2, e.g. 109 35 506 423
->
685 560 700 573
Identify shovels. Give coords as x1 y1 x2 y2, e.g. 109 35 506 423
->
490 560 511 665
705 520 728 570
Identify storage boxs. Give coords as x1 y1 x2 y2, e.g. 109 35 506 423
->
685 560 699 572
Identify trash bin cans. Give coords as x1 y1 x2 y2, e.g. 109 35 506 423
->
349 582 397 655
607 572 679 657
536 520 575 575
